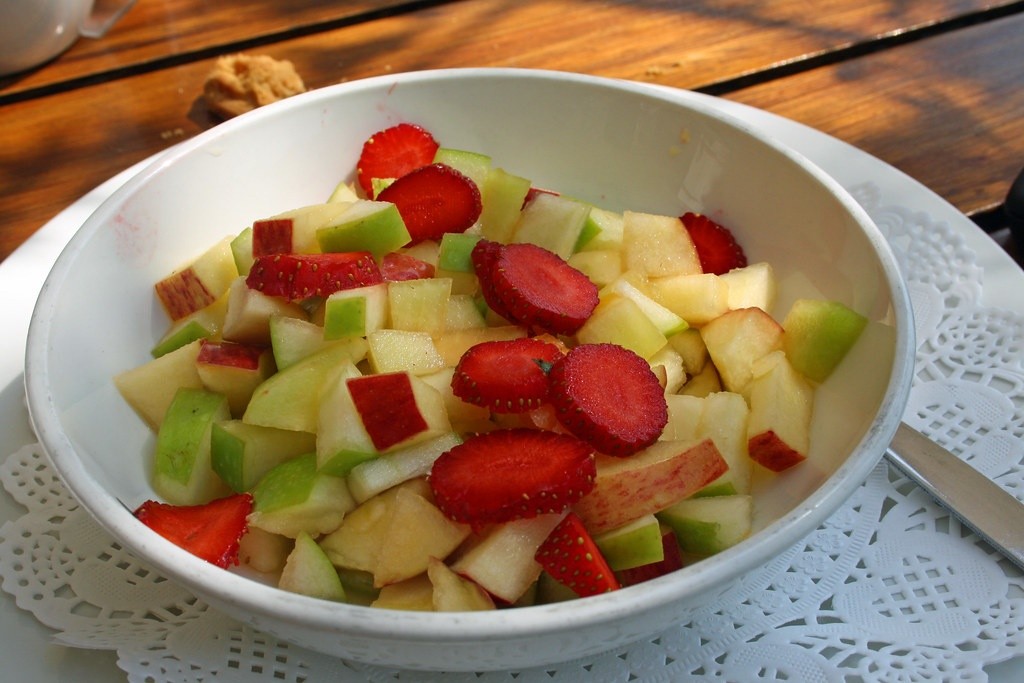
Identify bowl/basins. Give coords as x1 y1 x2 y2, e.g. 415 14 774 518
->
24 66 919 673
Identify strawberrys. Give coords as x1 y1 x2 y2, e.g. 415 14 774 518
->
134 125 749 597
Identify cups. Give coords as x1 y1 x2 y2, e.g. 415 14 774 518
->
0 0 140 80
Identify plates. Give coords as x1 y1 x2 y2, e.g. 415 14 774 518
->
0 94 1024 683
